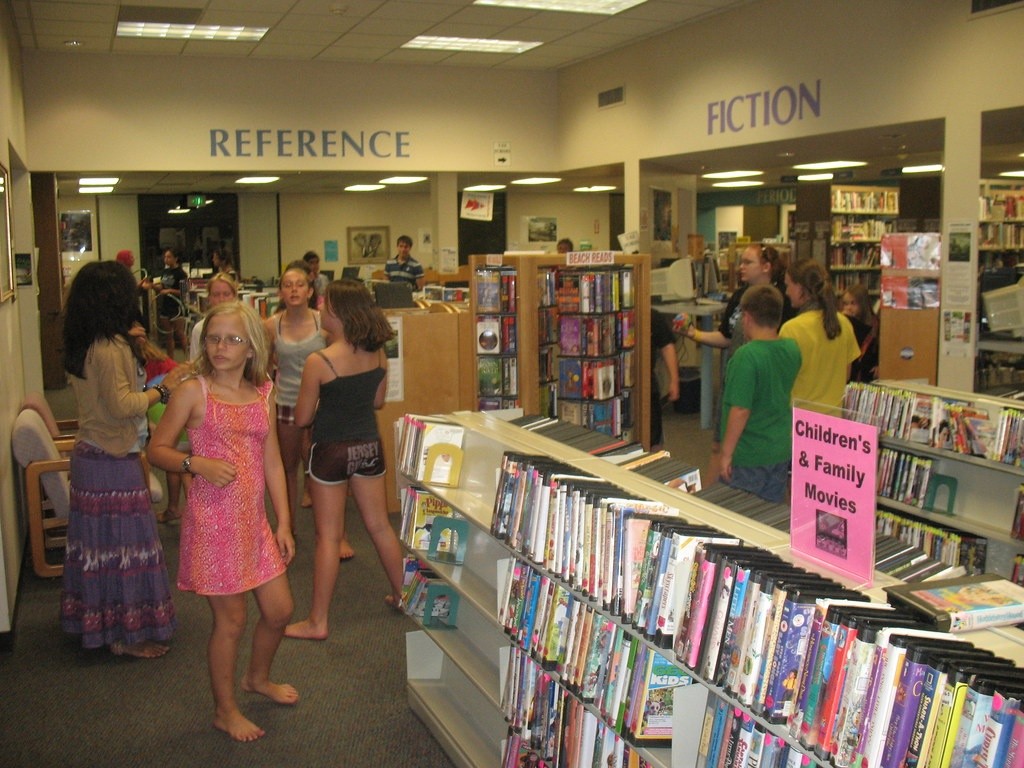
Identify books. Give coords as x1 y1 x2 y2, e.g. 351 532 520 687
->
395 412 464 617
418 267 633 438
490 449 1024 768
842 381 1024 469
834 190 1024 387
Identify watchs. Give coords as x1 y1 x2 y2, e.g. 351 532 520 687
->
182 454 196 478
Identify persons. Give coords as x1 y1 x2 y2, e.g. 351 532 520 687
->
213 247 235 274
262 251 404 639
153 246 188 358
383 236 425 289
116 248 150 289
557 238 575 252
651 245 879 504
58 259 189 657
189 271 238 360
130 320 191 523
146 300 299 741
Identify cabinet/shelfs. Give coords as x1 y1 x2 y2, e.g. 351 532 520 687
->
183 180 1024 768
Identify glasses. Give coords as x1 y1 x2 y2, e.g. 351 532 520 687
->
204 335 246 345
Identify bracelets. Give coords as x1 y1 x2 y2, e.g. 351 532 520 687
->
153 384 169 405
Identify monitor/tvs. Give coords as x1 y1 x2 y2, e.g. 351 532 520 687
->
650 257 721 301
319 270 334 282
341 266 361 281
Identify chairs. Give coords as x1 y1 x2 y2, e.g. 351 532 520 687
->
11 389 163 577
